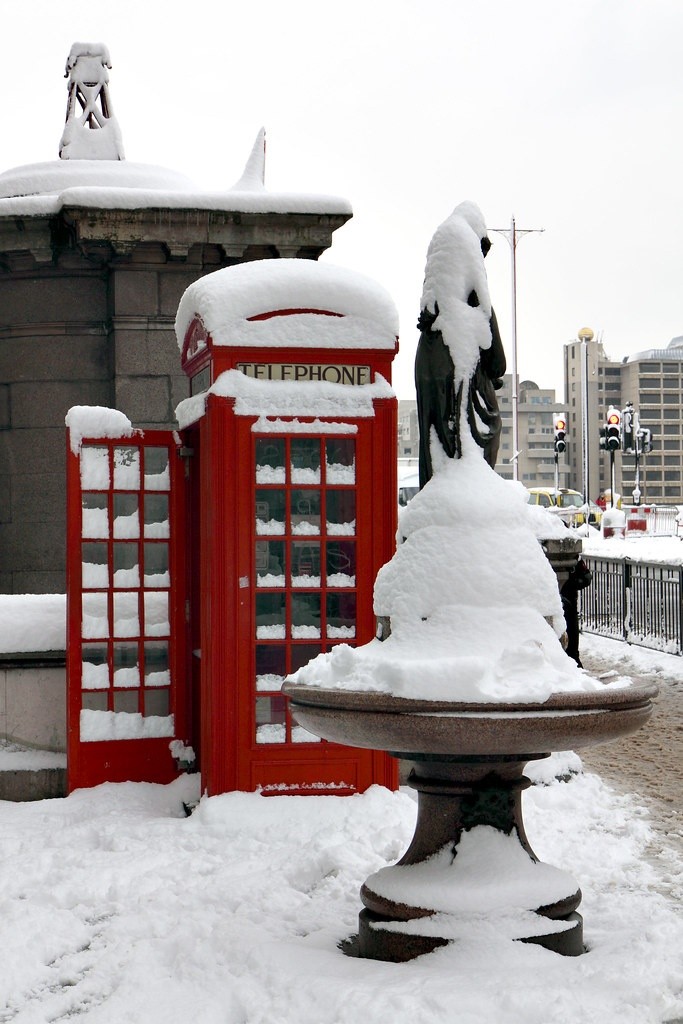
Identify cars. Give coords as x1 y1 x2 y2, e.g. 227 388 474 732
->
527 486 604 530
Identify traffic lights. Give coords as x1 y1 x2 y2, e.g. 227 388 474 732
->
598 423 609 450
608 411 621 451
555 415 567 452
639 428 654 455
